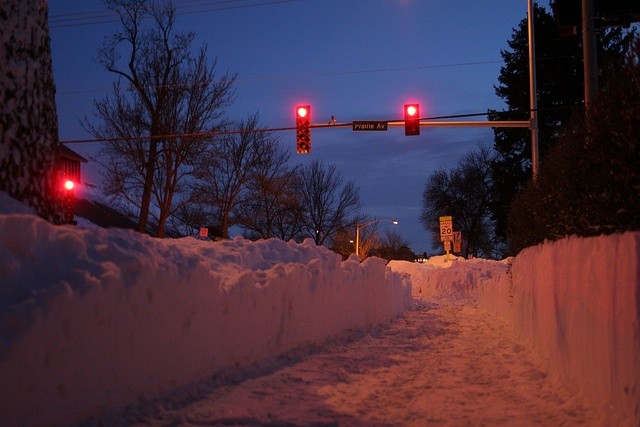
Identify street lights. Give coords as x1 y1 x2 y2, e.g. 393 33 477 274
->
356 218 399 256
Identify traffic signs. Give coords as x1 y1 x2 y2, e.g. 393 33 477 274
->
352 121 387 131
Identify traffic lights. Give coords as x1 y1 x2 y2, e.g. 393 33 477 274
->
404 103 421 136
295 104 312 154
